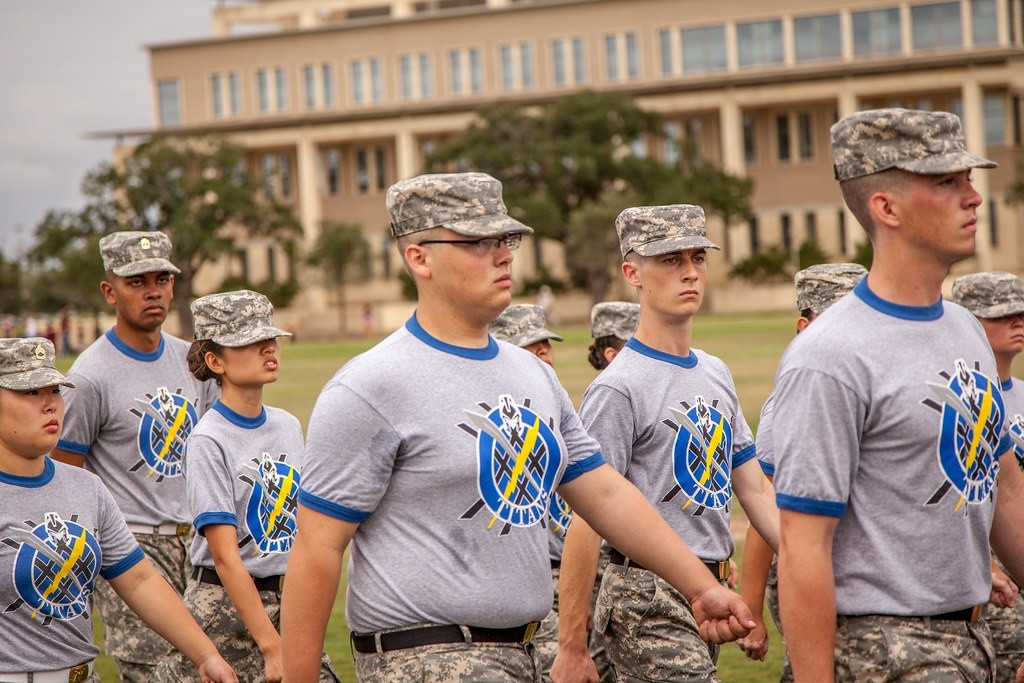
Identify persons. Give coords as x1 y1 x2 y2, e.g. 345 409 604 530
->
774 106 1024 683
488 301 643 682
278 171 757 683
951 272 1024 683
0 338 238 683
739 262 1018 683
551 204 781 683
48 230 223 683
148 289 345 683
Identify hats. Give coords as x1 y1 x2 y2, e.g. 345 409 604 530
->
486 304 564 350
795 263 869 313
950 269 1023 319
385 172 534 238
190 290 292 348
828 108 996 182
99 231 181 277
591 301 641 343
0 337 75 391
616 205 721 260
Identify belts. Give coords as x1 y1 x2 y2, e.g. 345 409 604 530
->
845 605 981 628
607 547 731 584
549 559 561 569
0 660 96 683
124 523 193 536
192 566 285 593
351 619 541 653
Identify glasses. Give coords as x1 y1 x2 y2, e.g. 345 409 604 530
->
417 232 522 257
